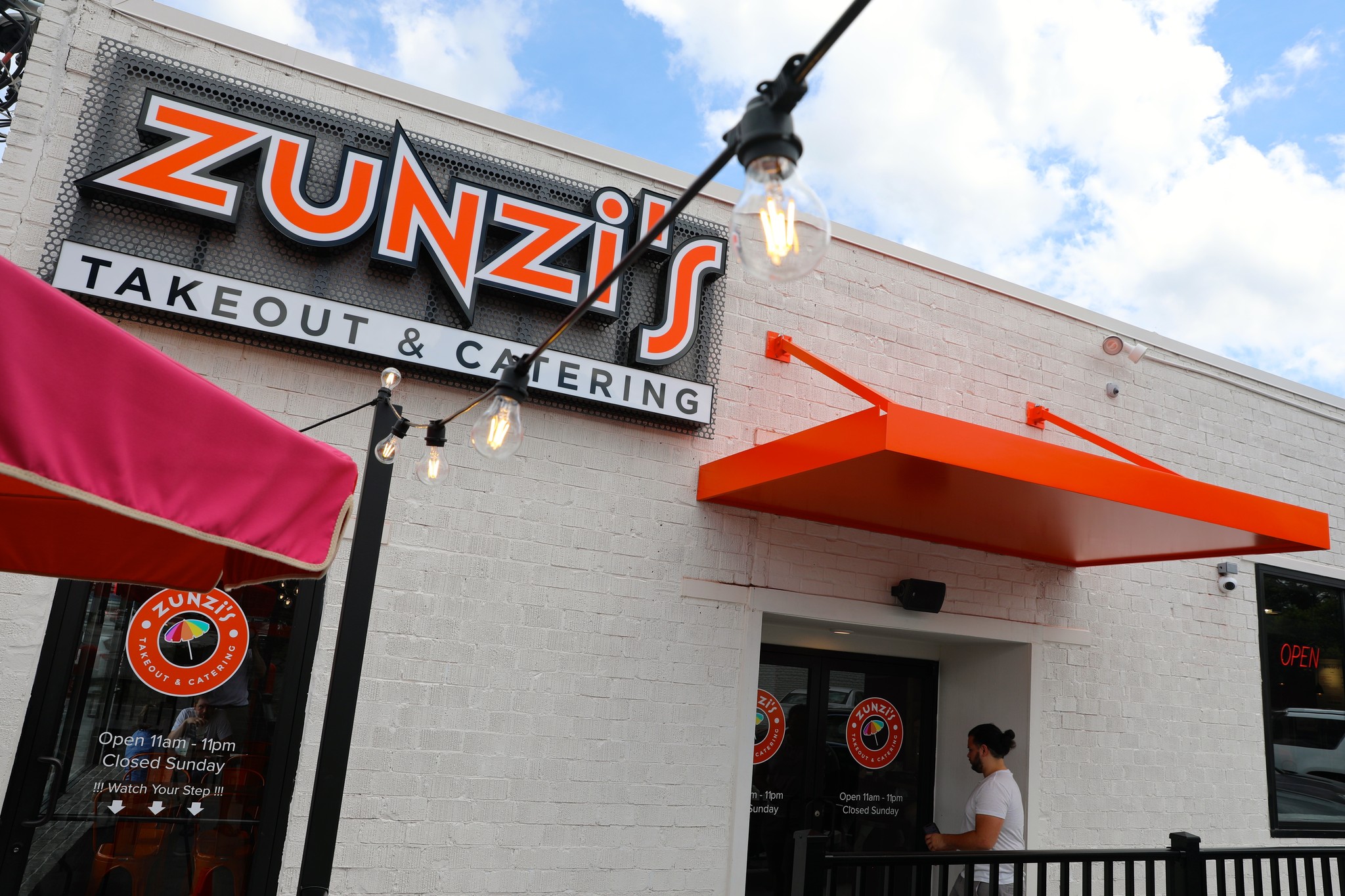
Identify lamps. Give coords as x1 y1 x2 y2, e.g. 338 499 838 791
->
1102 334 1149 364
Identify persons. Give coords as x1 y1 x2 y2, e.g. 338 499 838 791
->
126 639 265 782
925 724 1026 896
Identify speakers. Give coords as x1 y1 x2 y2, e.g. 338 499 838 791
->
897 579 946 614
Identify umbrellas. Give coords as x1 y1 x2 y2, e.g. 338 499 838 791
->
0 255 359 594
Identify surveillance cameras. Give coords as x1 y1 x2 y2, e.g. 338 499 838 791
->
1219 576 1237 592
